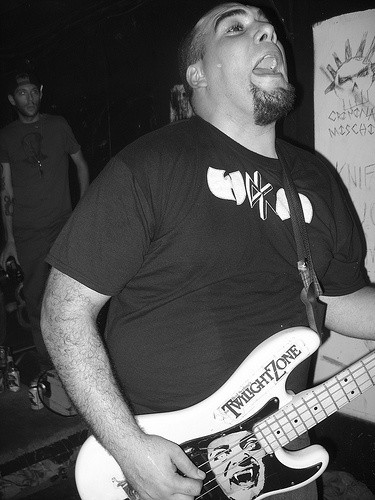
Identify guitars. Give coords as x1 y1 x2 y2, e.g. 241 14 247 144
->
73 324 375 500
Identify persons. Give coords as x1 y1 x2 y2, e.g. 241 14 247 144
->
40 2 375 500
0 70 89 371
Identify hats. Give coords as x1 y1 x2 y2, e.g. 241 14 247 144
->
9 72 43 90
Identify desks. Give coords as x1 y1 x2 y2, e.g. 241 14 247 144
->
0 383 88 478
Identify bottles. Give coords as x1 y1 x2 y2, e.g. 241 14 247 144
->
0 346 7 388
5 347 17 391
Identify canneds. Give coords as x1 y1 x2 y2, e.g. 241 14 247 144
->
29 384 44 410
6 368 20 392
